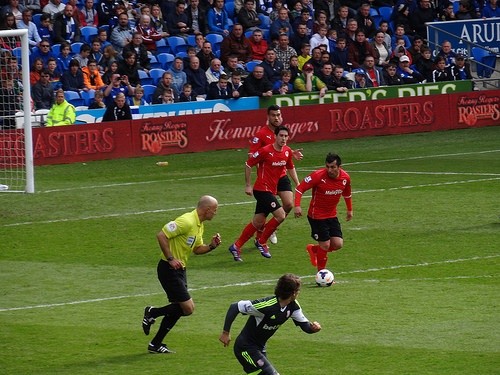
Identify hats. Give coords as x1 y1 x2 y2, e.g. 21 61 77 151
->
399 55 410 62
356 68 365 75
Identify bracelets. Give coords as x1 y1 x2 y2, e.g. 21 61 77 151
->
209 244 215 250
167 256 174 260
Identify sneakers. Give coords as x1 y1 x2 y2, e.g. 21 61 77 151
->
148 343 176 354
142 306 156 335
229 244 243 261
255 240 271 258
306 243 317 266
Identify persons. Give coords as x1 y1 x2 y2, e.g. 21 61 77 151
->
228 125 300 261
102 91 132 122
248 105 303 248
43 88 76 127
295 152 353 285
0 0 500 109
0 76 22 129
143 194 221 353
219 273 321 375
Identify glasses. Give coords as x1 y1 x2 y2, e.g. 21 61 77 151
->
42 44 50 47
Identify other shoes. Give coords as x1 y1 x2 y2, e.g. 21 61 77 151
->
269 232 277 244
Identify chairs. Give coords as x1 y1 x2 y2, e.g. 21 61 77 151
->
369 7 396 33
14 0 272 105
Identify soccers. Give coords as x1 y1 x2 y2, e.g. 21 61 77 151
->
315 268 333 287
